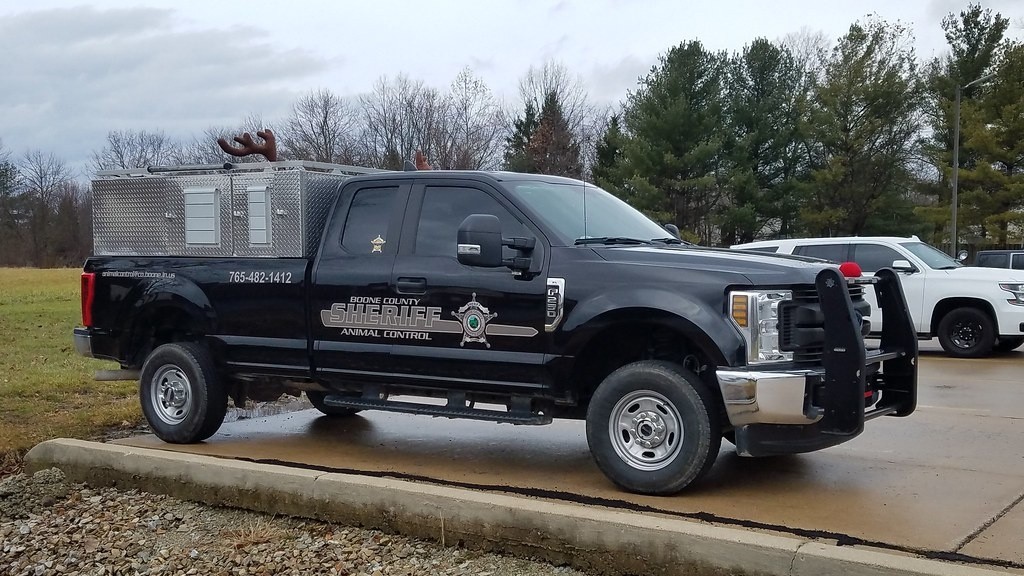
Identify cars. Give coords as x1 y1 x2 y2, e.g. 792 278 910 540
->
974 249 1024 269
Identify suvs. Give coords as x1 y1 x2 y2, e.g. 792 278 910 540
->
728 235 1024 359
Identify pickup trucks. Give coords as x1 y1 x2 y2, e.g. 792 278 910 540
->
72 168 921 498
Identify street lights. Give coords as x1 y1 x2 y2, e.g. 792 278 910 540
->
950 72 998 260
